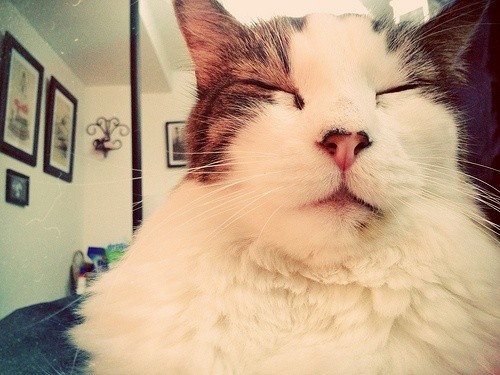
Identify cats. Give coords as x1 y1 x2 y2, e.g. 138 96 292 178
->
20 0 500 375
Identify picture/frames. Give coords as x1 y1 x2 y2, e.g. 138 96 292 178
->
165 121 190 168
1 29 45 167
6 168 29 205
42 75 78 183
